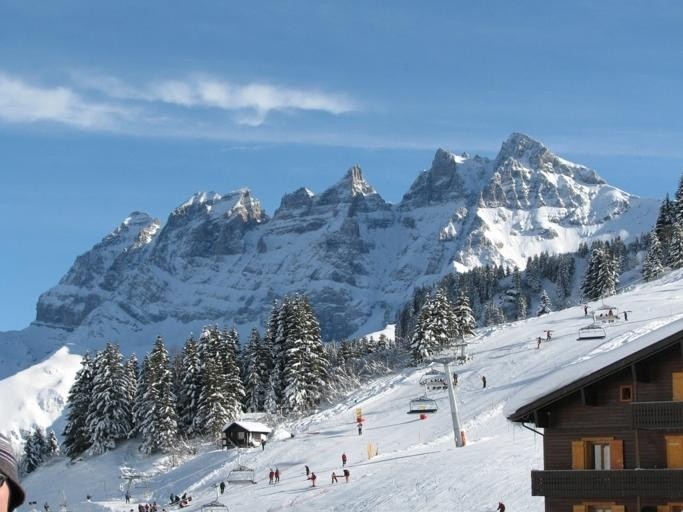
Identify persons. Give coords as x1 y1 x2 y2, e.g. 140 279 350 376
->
124 488 192 512
269 469 274 484
482 376 487 388
599 310 613 317
623 311 628 321
220 482 225 493
261 438 265 450
357 421 363 435
304 453 351 486
453 372 458 384
537 336 541 349
44 501 50 512
275 469 280 482
584 304 589 318
0 433 25 511
497 501 505 512
546 331 551 341
